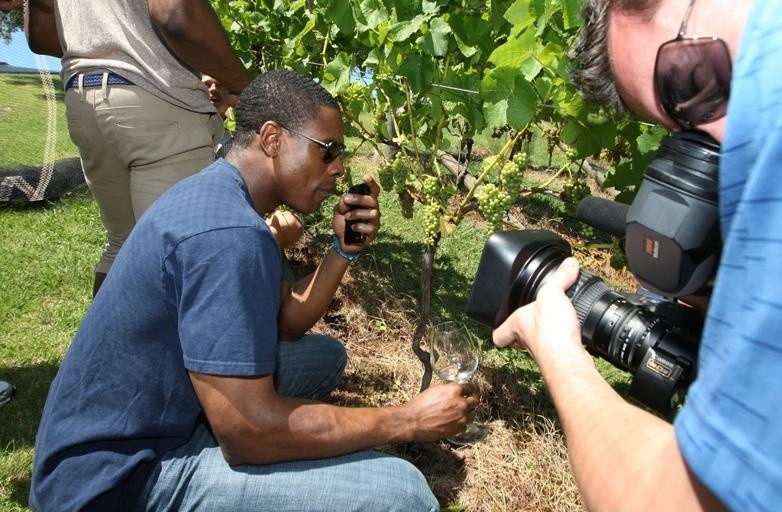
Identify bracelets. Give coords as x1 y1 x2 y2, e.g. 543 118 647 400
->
334 234 360 262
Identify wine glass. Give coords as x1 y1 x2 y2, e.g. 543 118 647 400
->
430 320 488 447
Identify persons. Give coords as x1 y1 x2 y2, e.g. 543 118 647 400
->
25 0 254 301
28 67 480 510
489 0 781 512
201 72 239 160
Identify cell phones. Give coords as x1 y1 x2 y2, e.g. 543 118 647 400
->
343 182 372 243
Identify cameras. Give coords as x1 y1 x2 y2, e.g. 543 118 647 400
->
464 129 726 413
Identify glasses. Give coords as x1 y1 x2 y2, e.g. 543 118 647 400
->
653 1 732 129
255 123 346 163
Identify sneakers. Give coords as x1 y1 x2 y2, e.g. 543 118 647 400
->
0 381 16 407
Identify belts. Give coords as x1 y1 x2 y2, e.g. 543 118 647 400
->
64 73 134 88
